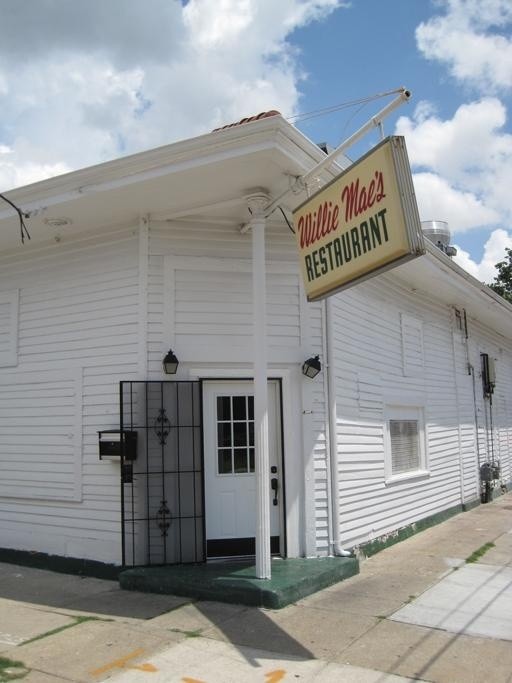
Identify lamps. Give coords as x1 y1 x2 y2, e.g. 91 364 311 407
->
162 348 179 374
301 355 321 380
